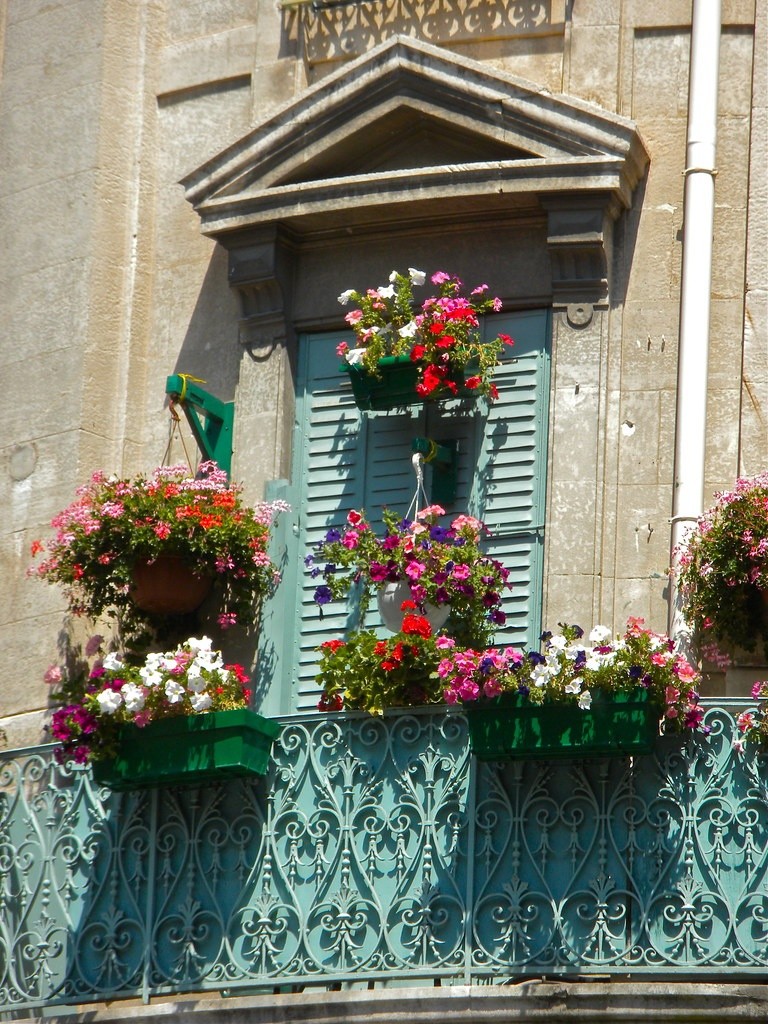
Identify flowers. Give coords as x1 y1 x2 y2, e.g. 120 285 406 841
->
335 267 513 402
24 460 768 771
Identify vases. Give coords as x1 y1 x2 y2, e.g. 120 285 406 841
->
340 353 483 412
463 685 661 762
87 710 281 792
129 555 216 614
377 579 452 637
